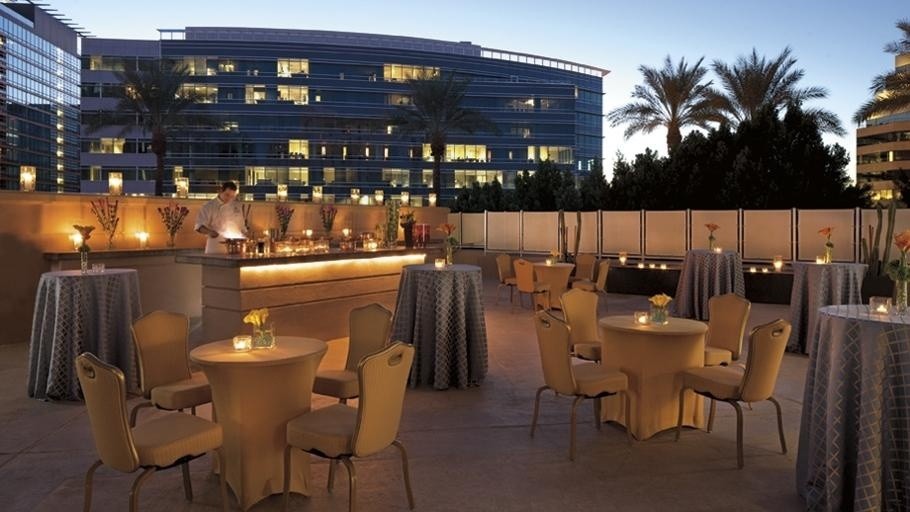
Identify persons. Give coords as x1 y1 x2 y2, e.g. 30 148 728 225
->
193 181 251 255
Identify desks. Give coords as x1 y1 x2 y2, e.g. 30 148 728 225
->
785 262 869 356
598 315 709 441
26 268 143 401
387 263 488 391
189 336 328 512
743 270 794 305
796 305 910 512
668 248 745 321
607 263 682 299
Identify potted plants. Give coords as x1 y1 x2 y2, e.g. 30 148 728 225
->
862 201 897 305
557 208 581 277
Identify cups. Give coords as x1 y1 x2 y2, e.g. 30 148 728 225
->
638 262 667 269
177 178 189 199
249 224 386 256
108 172 123 196
749 266 769 273
870 296 893 314
435 258 445 268
20 166 37 192
635 310 648 324
232 334 252 353
92 263 107 273
816 255 825 263
278 185 436 207
226 238 246 256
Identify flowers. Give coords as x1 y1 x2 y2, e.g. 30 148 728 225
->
434 221 457 236
819 224 835 248
703 221 720 243
320 202 337 230
550 248 559 257
156 202 191 233
90 196 122 238
242 307 270 330
276 203 295 234
648 293 672 312
73 224 95 240
892 230 910 302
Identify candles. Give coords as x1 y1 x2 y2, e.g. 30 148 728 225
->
660 263 666 269
816 259 826 264
877 304 888 314
619 256 626 265
278 244 328 253
435 262 443 268
649 264 655 268
364 242 377 252
762 267 768 273
638 263 644 269
750 267 756 273
639 316 648 324
235 340 246 349
714 248 722 254
774 261 783 268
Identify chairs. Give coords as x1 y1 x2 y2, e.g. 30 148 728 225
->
704 292 753 411
674 318 792 471
496 254 611 315
558 287 602 365
281 340 416 512
312 303 396 464
74 351 229 512
130 309 217 423
529 310 632 461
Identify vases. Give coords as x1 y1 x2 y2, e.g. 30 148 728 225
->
104 239 113 249
447 238 453 265
253 328 274 348
895 249 908 316
80 253 88 274
77 238 89 253
655 309 669 325
166 234 176 248
826 235 832 263
709 231 713 250
